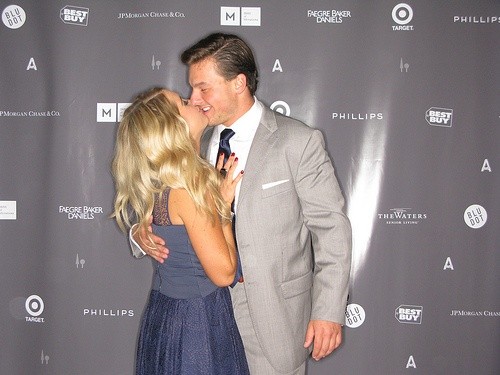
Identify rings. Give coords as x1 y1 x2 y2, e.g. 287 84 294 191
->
219 167 227 176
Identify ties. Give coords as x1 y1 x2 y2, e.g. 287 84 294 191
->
214 128 242 289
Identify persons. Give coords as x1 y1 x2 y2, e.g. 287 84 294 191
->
127 32 352 375
109 88 249 375
